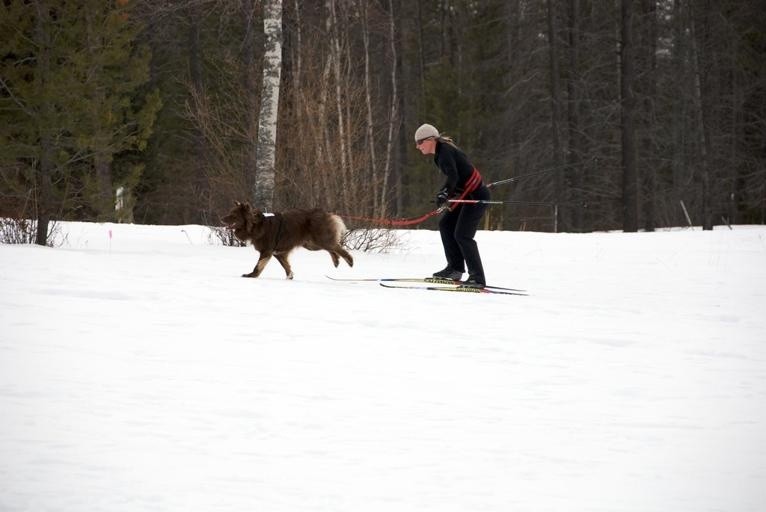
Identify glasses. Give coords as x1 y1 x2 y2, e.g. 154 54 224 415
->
416 136 435 146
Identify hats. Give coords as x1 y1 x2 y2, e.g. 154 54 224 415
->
414 123 440 142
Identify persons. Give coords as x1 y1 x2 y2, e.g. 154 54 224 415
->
414 123 491 289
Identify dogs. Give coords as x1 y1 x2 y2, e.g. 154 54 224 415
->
220 200 354 280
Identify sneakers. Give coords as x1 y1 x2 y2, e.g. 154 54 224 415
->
459 275 486 288
433 264 462 281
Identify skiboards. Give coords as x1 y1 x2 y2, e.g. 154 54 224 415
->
324 276 528 295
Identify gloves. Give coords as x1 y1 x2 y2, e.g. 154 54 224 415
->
436 188 451 207
